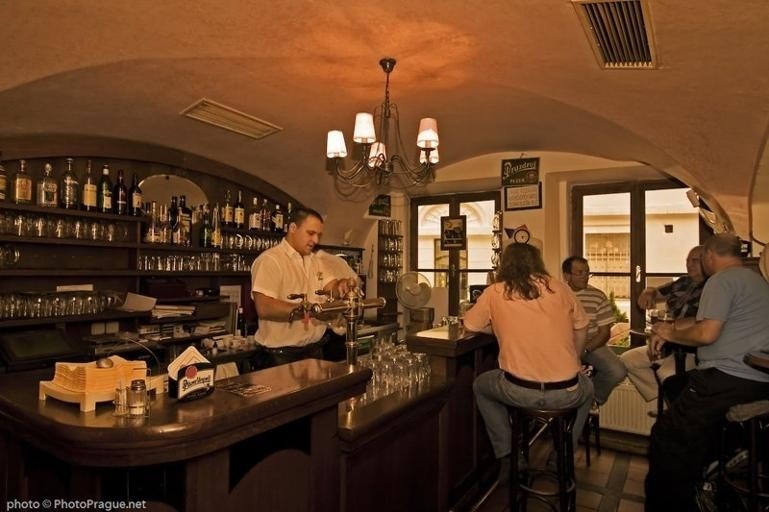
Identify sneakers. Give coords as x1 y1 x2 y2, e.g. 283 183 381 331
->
499 451 528 484
647 408 669 419
547 450 569 474
589 401 599 415
706 446 750 481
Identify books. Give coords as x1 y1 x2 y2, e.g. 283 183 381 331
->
134 303 224 342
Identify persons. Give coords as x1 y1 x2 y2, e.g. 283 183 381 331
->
644 231 769 510
311 243 363 361
619 246 704 415
460 242 596 484
562 255 627 405
251 206 350 370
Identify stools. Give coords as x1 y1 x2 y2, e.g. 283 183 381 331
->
506 406 600 512
701 396 768 512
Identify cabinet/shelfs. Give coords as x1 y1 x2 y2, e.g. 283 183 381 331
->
0 134 309 376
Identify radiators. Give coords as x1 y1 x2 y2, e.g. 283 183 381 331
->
597 377 667 437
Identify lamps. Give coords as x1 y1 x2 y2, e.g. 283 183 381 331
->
325 58 440 200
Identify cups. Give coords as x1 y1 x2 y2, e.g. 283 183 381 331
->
0 294 106 320
139 249 251 272
0 211 130 243
215 335 257 350
115 387 128 415
347 340 433 402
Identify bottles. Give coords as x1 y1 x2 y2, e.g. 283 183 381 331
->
236 306 248 338
0 157 142 217
144 184 294 251
128 379 148 417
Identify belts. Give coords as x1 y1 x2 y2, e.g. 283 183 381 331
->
504 371 578 390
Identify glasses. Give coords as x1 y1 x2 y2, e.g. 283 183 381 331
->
571 270 589 275
